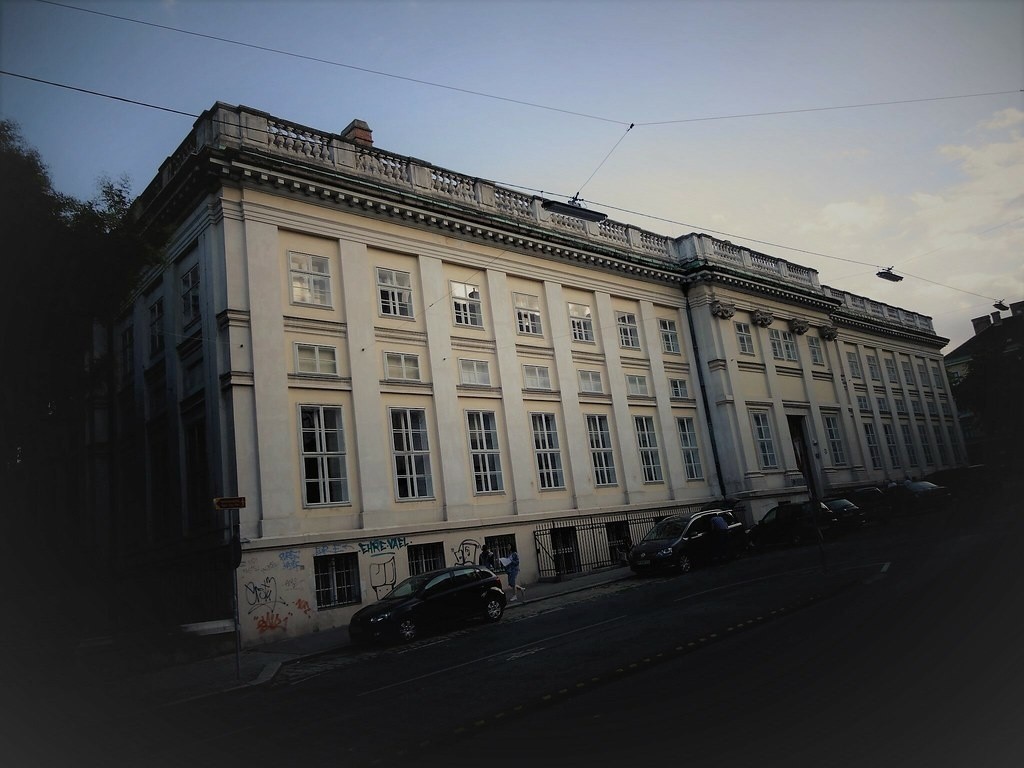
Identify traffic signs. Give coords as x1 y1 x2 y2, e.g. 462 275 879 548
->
214 496 244 510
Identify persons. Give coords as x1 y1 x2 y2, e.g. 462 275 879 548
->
498 543 526 602
478 544 496 570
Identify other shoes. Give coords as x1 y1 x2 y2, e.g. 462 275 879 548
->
521 587 525 592
510 596 517 601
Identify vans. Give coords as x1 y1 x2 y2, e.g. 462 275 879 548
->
627 508 748 575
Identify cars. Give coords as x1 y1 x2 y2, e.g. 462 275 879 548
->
923 463 991 502
350 564 506 644
870 480 953 525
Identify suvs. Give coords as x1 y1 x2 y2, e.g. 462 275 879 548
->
748 499 864 550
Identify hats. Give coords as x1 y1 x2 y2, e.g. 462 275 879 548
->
481 544 490 551
